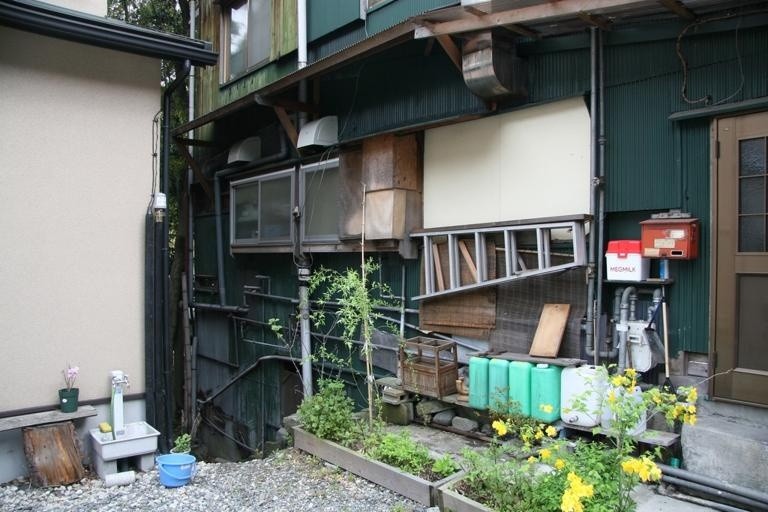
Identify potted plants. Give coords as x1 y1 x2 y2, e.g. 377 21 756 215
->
288 381 462 508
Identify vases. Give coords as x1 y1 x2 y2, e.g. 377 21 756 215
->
58 388 79 413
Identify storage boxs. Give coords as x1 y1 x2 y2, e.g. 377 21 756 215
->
603 239 651 282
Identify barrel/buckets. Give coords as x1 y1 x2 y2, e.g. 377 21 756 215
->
155 452 196 486
468 354 649 436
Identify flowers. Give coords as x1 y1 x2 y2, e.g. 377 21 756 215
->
62 366 80 392
459 366 697 511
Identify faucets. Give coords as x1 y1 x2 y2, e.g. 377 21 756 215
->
116 375 131 389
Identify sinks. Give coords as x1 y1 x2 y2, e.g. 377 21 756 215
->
88 421 161 461
